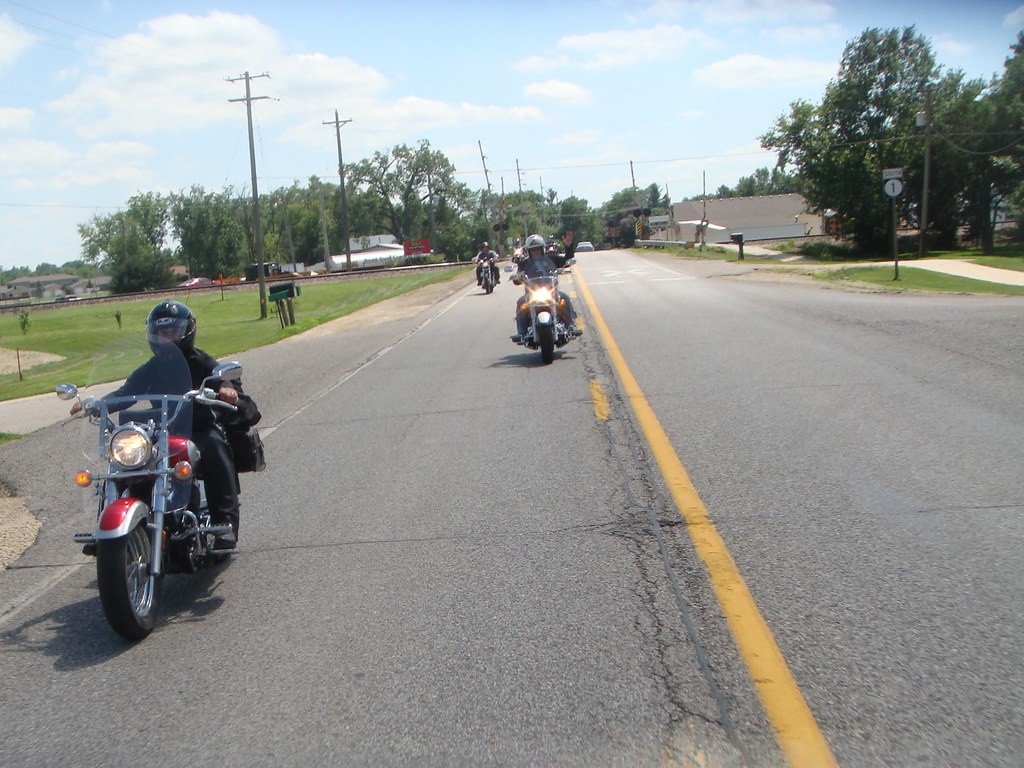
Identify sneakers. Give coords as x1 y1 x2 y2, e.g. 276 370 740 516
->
569 325 583 335
512 334 526 342
215 532 236 548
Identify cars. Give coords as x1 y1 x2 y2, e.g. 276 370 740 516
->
575 242 594 252
176 278 215 288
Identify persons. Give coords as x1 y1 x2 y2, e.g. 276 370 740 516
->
508 230 583 341
475 242 501 285
70 300 261 556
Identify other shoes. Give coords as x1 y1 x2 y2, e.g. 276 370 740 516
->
496 280 501 284
477 282 482 286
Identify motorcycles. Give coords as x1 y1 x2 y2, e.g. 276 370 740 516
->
472 254 500 294
546 243 560 255
57 333 265 642
510 244 525 263
504 256 576 365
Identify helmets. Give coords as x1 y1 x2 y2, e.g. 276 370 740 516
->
481 241 490 247
146 301 197 357
525 234 546 256
